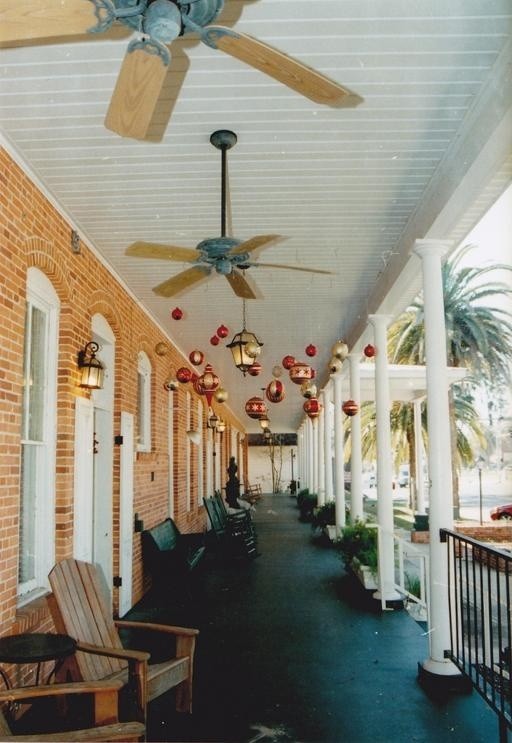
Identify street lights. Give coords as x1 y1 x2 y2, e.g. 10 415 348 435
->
476 454 484 527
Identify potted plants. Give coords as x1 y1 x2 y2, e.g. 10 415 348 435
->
401 568 428 623
309 500 352 541
334 520 392 589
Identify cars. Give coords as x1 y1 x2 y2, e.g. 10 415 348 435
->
488 500 512 522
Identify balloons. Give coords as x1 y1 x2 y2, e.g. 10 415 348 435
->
153 304 376 417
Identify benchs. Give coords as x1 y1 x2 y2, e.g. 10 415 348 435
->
140 516 218 606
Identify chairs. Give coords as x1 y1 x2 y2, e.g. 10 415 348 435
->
201 480 266 561
39 555 202 742
1 677 151 742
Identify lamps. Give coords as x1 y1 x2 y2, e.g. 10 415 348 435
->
224 263 264 378
258 387 273 445
215 418 227 435
76 340 107 393
207 411 220 432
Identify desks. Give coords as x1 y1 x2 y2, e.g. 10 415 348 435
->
0 629 79 688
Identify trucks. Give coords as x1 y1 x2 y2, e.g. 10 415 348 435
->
367 463 410 489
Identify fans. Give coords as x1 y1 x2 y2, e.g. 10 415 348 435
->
122 128 343 302
0 0 354 145
